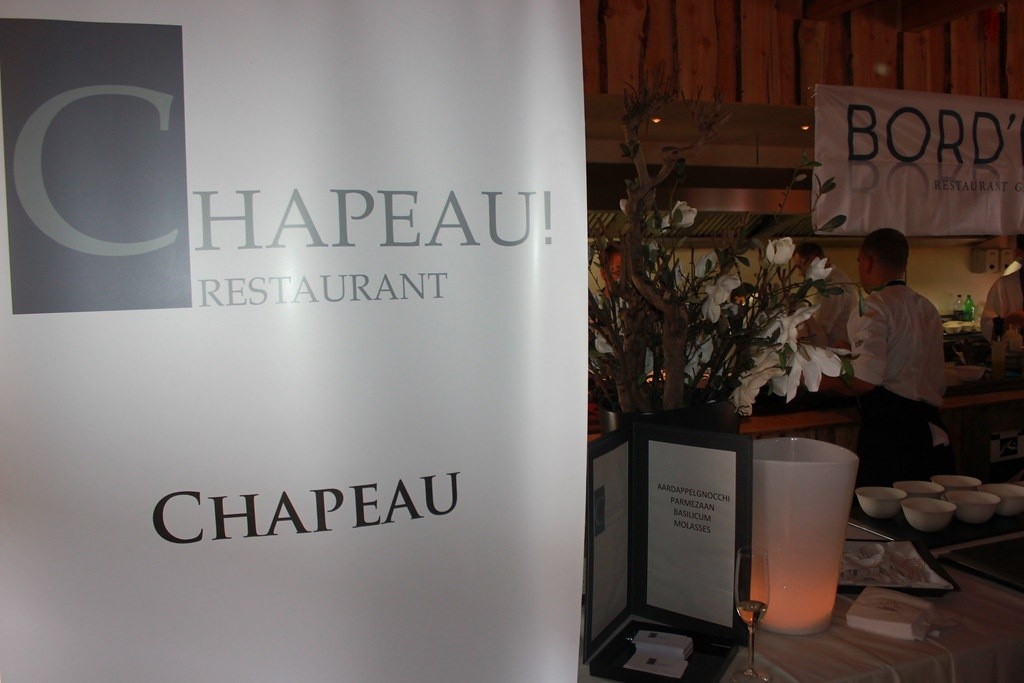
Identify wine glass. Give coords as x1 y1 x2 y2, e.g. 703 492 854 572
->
730 546 773 683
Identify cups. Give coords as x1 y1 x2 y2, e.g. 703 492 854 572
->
746 437 860 635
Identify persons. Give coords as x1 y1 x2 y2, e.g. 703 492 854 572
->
793 241 859 358
981 234 1024 372
588 241 654 391
730 282 769 363
801 227 951 488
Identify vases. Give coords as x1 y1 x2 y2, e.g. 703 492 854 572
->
601 388 750 436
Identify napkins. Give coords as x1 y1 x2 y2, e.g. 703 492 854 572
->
846 587 936 641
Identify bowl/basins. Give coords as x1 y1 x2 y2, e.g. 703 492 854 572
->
977 483 1024 515
893 481 945 497
931 475 983 492
855 487 907 518
942 320 981 333
958 365 986 382
901 496 957 531
942 490 1002 523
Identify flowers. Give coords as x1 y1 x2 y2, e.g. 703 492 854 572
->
588 0 862 420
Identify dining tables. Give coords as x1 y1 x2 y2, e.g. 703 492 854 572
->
577 481 1024 683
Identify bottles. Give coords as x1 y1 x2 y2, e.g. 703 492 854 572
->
991 325 1022 379
962 295 975 322
953 294 964 321
